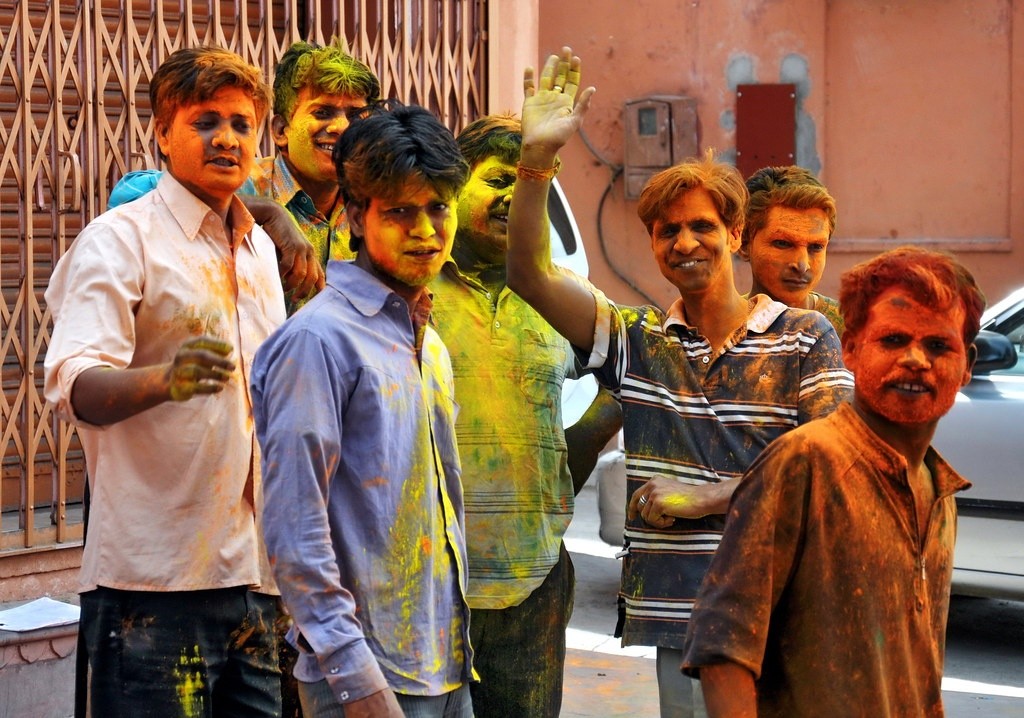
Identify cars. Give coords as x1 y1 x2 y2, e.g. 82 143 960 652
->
598 288 1024 601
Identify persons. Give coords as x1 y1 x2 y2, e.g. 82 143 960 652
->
505 45 856 718
249 104 481 718
424 113 624 718
43 45 304 718
735 165 849 341
106 41 381 319
678 243 987 718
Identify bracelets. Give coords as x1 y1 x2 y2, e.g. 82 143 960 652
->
516 155 562 185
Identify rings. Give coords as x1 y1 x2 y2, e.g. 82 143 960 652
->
639 495 647 506
553 85 562 91
